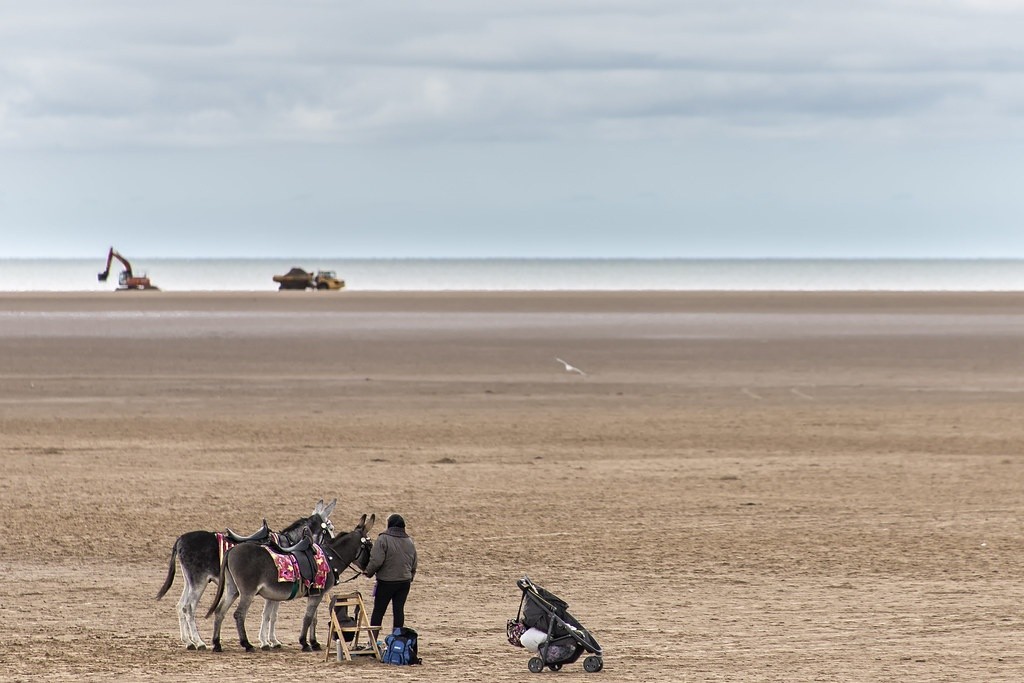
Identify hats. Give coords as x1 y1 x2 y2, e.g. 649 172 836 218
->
386 513 406 528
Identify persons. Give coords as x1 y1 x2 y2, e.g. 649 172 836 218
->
358 514 417 651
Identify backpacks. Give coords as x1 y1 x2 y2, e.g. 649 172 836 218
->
379 627 422 665
327 593 360 642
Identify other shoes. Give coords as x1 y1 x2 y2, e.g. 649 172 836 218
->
359 645 374 654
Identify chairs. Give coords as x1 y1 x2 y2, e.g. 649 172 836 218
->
325 589 382 662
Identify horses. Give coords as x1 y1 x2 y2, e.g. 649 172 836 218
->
205 512 375 653
153 498 340 652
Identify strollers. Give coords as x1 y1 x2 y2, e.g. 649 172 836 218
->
506 577 604 673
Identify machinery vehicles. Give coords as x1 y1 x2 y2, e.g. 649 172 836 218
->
273 268 345 292
97 247 161 291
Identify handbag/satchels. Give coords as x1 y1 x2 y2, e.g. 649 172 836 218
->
519 626 553 654
506 619 528 648
